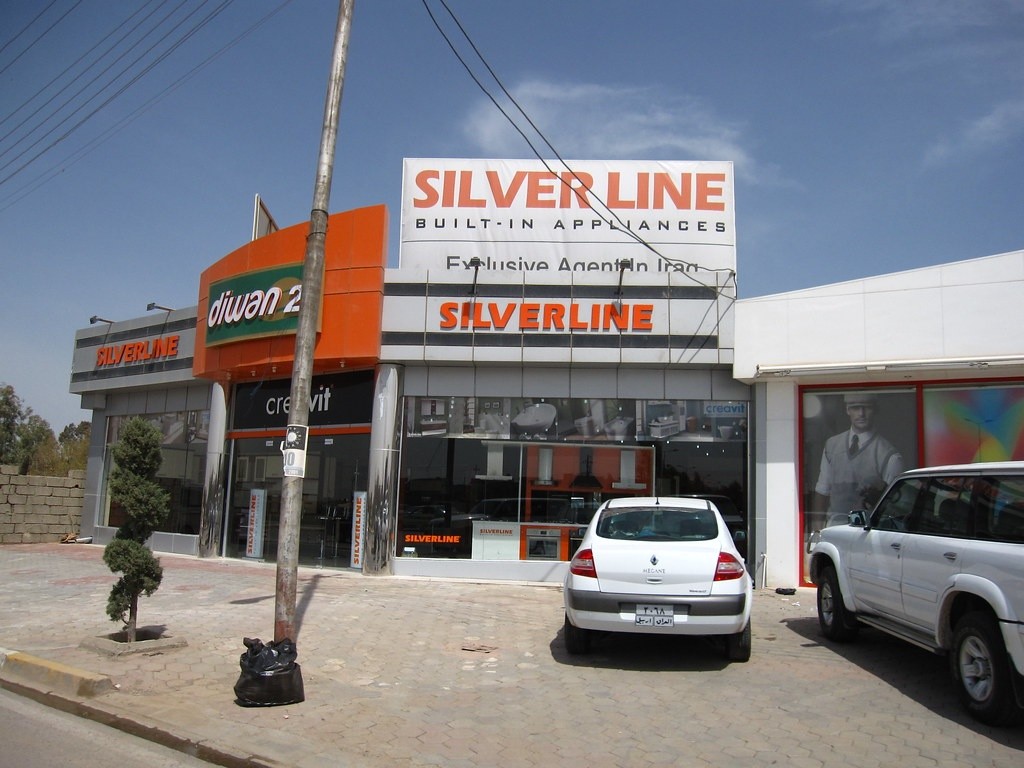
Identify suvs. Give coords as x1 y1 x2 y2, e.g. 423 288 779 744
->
806 460 1024 720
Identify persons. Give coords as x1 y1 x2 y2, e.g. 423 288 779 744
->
810 394 905 533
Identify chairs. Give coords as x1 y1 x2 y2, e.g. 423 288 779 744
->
938 498 969 533
995 504 1024 542
300 502 351 560
678 519 704 538
613 521 637 533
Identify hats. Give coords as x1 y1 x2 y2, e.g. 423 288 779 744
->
843 392 877 407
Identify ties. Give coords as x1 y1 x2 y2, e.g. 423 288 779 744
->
847 435 859 454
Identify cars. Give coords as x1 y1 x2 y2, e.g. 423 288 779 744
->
564 497 752 664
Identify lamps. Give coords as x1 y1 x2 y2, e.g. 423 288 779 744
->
468 257 482 295
146 303 173 312
89 316 113 323
616 260 629 314
